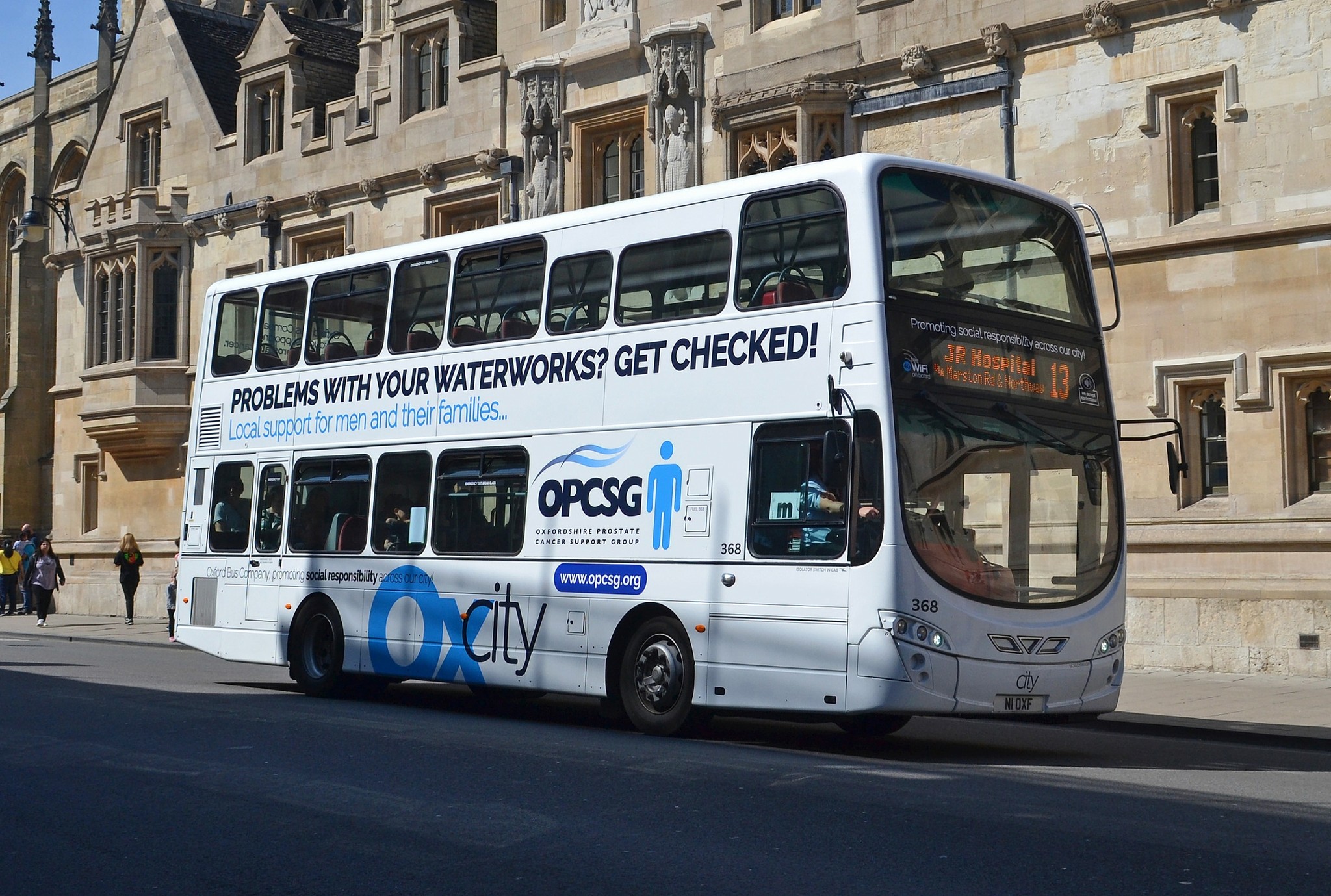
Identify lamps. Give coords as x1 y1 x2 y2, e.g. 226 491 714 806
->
14 193 73 243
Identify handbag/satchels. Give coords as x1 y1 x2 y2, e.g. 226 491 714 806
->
17 573 23 585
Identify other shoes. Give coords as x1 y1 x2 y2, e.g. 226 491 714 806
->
18 611 33 615
125 616 133 625
0 612 5 616
18 607 24 610
169 636 175 642
43 623 48 626
166 626 168 629
6 611 15 616
36 619 44 626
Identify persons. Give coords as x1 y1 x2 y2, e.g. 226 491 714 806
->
167 568 178 642
212 472 522 555
796 452 880 556
21 524 36 611
113 534 144 625
174 537 181 568
0 539 24 617
12 532 33 556
525 134 557 219
23 538 66 627
659 104 694 192
18 531 34 614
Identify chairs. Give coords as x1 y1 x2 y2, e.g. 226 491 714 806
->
209 498 376 554
212 266 818 550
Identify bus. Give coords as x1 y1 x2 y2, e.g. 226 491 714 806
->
170 155 1192 737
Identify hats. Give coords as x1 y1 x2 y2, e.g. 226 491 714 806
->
45 531 52 540
22 524 30 532
